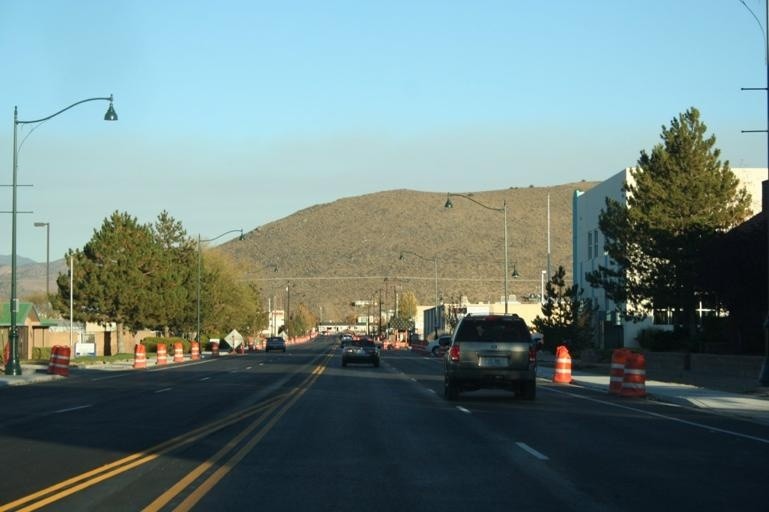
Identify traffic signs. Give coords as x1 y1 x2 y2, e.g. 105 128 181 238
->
243 262 308 348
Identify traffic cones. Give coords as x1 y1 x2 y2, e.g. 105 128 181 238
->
47 343 71 376
552 346 574 387
610 347 647 399
133 340 220 369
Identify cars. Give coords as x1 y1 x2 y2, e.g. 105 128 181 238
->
265 336 286 354
438 313 538 401
342 338 381 367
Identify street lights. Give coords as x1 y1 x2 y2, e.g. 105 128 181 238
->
197 227 245 359
4 94 117 376
445 190 509 313
33 220 55 321
368 255 396 353
399 250 441 353
